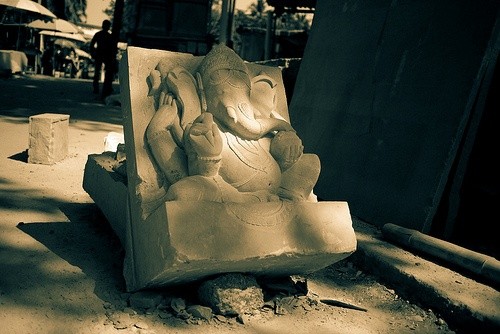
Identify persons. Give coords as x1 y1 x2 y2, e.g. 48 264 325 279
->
86 19 118 104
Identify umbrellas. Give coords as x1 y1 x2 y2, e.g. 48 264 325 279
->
24 15 93 77
0 0 58 29
